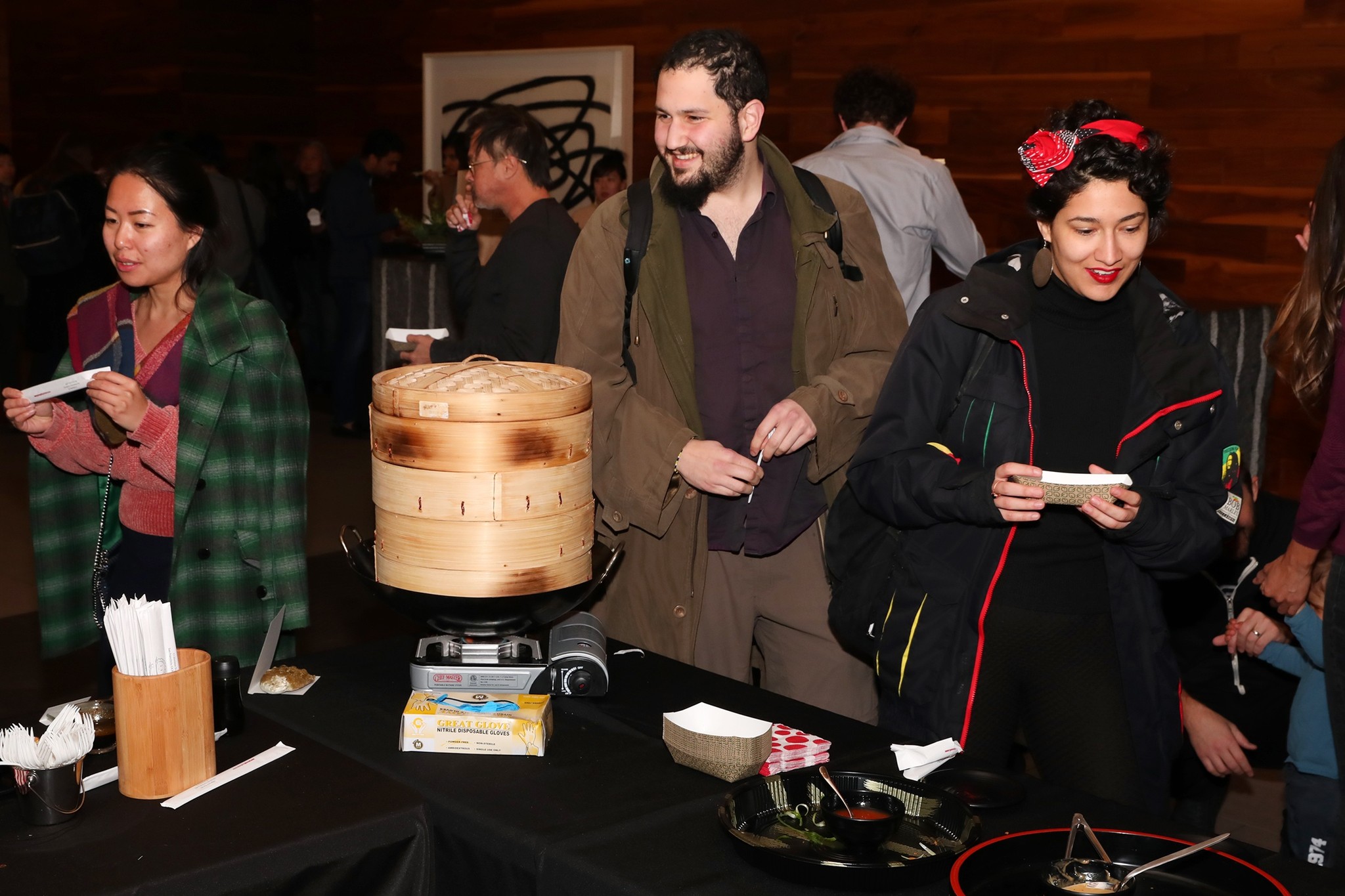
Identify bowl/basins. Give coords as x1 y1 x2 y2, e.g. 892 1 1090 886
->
74 699 116 749
820 789 905 846
1042 857 1136 896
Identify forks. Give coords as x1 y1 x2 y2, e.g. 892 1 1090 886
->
0 704 95 770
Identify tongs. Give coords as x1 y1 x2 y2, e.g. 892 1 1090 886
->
1064 813 1112 864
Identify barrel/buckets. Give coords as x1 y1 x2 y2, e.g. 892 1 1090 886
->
11 739 85 826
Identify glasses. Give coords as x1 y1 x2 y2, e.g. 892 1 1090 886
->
467 158 527 178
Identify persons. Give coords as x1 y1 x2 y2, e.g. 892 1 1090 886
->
847 98 1244 816
1252 139 1345 869
791 67 986 327
1256 549 1341 862
326 128 405 437
0 131 109 392
556 27 908 727
2 140 307 668
1152 467 1294 833
400 104 581 363
572 150 627 229
187 132 267 289
275 138 336 392
425 131 507 265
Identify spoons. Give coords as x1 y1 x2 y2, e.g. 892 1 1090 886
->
1086 832 1231 892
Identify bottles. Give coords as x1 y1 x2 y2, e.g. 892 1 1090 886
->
211 655 245 734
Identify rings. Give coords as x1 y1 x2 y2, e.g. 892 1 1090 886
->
991 481 1001 498
1252 630 1260 636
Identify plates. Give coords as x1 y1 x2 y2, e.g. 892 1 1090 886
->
920 769 1014 807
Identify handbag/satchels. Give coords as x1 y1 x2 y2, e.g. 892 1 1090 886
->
826 330 992 668
